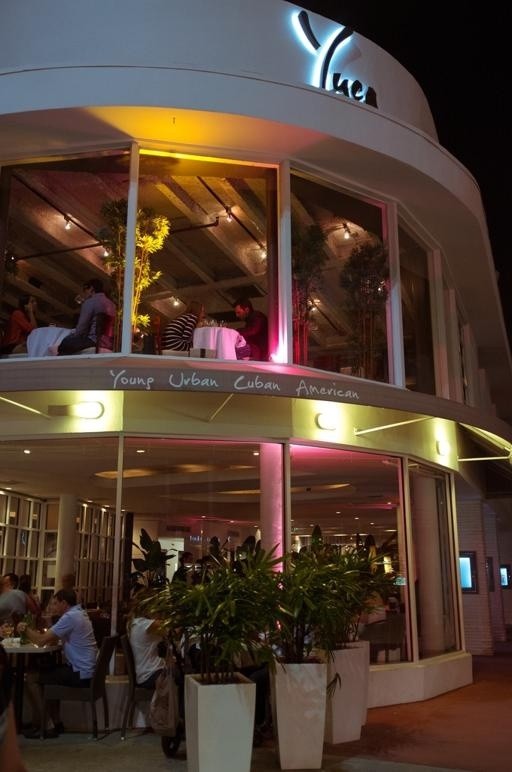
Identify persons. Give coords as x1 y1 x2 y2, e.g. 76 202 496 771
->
160 300 207 358
55 278 118 357
117 537 390 714
1 574 37 623
3 572 21 590
16 586 100 740
1 644 31 772
231 295 269 362
1 294 49 354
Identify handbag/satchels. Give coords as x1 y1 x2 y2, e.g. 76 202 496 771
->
148 642 180 737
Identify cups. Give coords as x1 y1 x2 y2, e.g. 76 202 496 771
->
5 619 15 635
198 318 230 327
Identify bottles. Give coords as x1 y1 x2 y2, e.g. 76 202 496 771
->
20 606 34 644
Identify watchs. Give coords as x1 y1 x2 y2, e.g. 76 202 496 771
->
19 624 29 634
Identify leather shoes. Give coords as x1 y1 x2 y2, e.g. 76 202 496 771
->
23 725 57 738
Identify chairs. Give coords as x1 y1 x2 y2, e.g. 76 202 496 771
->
43 633 155 741
152 313 189 356
78 312 115 355
